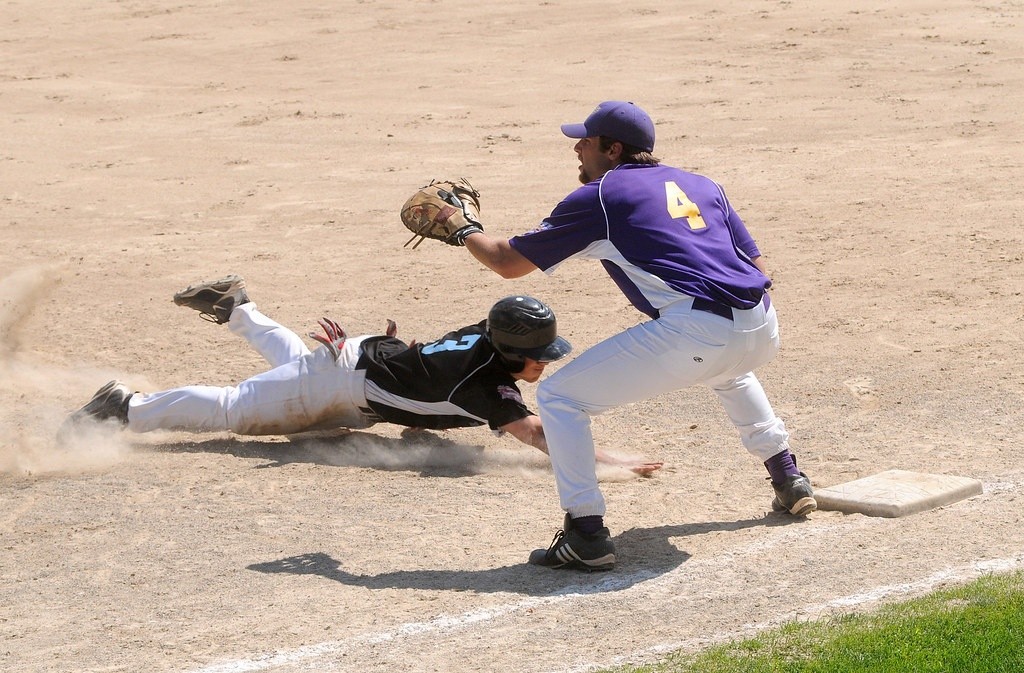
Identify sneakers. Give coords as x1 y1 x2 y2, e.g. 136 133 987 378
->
173 275 249 325
529 513 615 570
58 380 138 441
765 454 817 518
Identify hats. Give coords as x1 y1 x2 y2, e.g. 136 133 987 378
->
561 101 655 151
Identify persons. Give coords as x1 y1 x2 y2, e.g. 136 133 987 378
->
400 102 817 573
57 275 663 480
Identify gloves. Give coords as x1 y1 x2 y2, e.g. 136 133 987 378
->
309 317 347 362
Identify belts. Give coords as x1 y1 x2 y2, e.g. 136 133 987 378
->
354 353 388 423
692 296 735 321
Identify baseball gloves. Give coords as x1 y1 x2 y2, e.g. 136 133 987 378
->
400 176 485 250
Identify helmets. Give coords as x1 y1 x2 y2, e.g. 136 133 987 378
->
486 296 572 373
